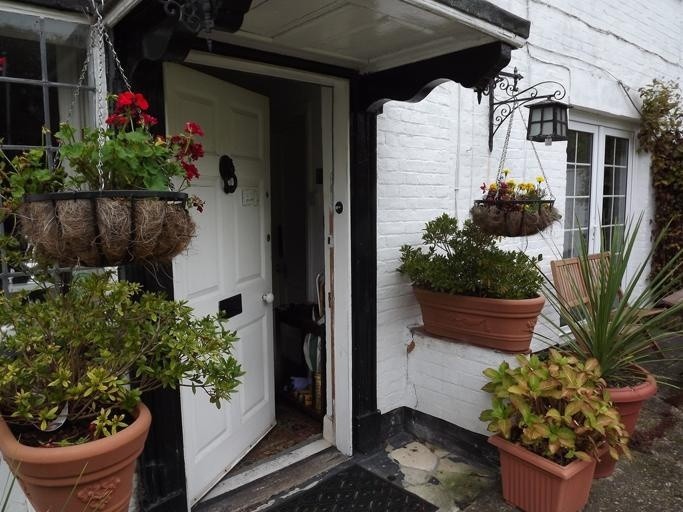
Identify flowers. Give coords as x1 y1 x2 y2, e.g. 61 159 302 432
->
479 169 545 202
0 91 205 214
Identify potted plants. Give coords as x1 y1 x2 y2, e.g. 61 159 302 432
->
0 276 245 512
516 208 682 477
396 212 544 353
478 347 632 512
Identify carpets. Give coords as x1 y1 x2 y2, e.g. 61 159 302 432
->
258 464 440 512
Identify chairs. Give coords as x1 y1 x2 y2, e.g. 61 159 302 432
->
550 251 667 367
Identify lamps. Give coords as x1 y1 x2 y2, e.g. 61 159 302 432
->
484 66 575 152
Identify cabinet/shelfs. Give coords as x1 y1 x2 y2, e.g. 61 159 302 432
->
272 303 326 422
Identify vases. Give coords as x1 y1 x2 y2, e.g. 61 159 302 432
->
471 199 561 236
16 191 193 266
